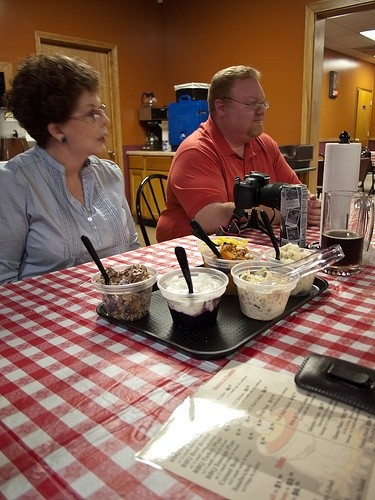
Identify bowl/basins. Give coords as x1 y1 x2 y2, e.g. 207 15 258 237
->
91 264 158 322
196 236 263 296
230 261 300 321
264 247 320 297
156 267 229 330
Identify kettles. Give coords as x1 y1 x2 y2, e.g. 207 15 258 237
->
146 132 159 145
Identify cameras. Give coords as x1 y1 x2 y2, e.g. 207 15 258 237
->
232 170 291 212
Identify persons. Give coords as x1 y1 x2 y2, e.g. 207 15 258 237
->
0 52 141 285
155 65 322 243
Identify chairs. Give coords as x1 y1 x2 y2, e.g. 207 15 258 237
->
135 174 168 246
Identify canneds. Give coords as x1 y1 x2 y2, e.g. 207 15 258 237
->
280 184 309 248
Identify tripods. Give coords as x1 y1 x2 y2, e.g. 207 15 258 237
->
241 206 280 241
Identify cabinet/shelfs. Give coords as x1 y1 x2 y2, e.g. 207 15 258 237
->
128 155 173 228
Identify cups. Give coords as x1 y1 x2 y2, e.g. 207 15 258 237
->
319 189 375 276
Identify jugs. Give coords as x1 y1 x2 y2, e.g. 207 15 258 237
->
141 92 157 107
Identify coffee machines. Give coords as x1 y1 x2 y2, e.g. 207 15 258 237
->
138 106 169 151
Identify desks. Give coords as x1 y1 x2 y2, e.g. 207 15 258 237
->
1 221 375 500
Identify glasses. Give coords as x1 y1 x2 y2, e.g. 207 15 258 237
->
220 96 271 111
68 104 107 123
